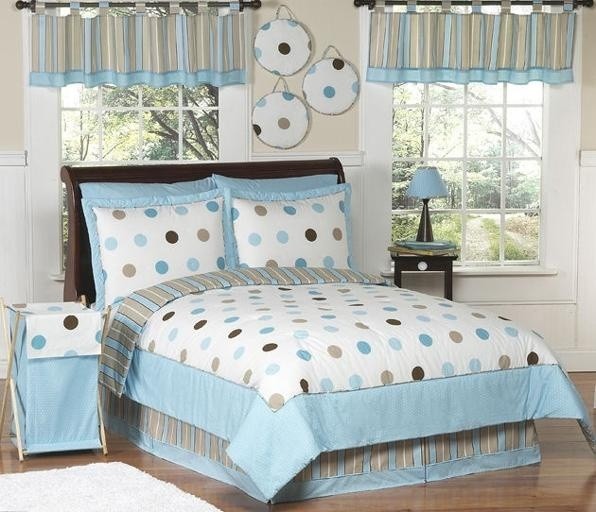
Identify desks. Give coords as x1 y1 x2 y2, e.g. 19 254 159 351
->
390 254 458 301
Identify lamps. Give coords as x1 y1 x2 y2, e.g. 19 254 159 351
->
403 165 450 243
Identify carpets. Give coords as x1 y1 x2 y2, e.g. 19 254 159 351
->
0 459 222 512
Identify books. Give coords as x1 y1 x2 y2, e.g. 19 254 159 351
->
393 239 457 251
387 246 457 256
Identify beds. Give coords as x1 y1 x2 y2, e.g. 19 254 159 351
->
59 157 542 504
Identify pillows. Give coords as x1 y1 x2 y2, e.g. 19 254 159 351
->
76 172 356 311
